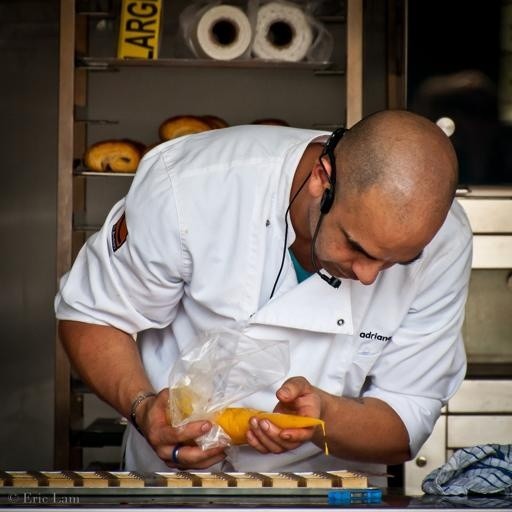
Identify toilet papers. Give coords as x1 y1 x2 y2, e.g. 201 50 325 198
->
253 1 314 62
197 4 252 62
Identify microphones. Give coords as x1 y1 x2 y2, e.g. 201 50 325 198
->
310 214 341 288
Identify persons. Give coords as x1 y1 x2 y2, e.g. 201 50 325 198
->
53 108 474 488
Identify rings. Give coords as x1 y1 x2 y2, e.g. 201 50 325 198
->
172 443 183 465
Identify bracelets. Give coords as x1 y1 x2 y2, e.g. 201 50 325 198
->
131 392 157 436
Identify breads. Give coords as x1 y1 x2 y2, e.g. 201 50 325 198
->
161 118 210 140
85 140 140 171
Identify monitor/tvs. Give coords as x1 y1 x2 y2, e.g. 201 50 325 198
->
404 0 512 198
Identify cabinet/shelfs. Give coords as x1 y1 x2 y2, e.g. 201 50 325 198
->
52 1 365 474
385 186 512 498
